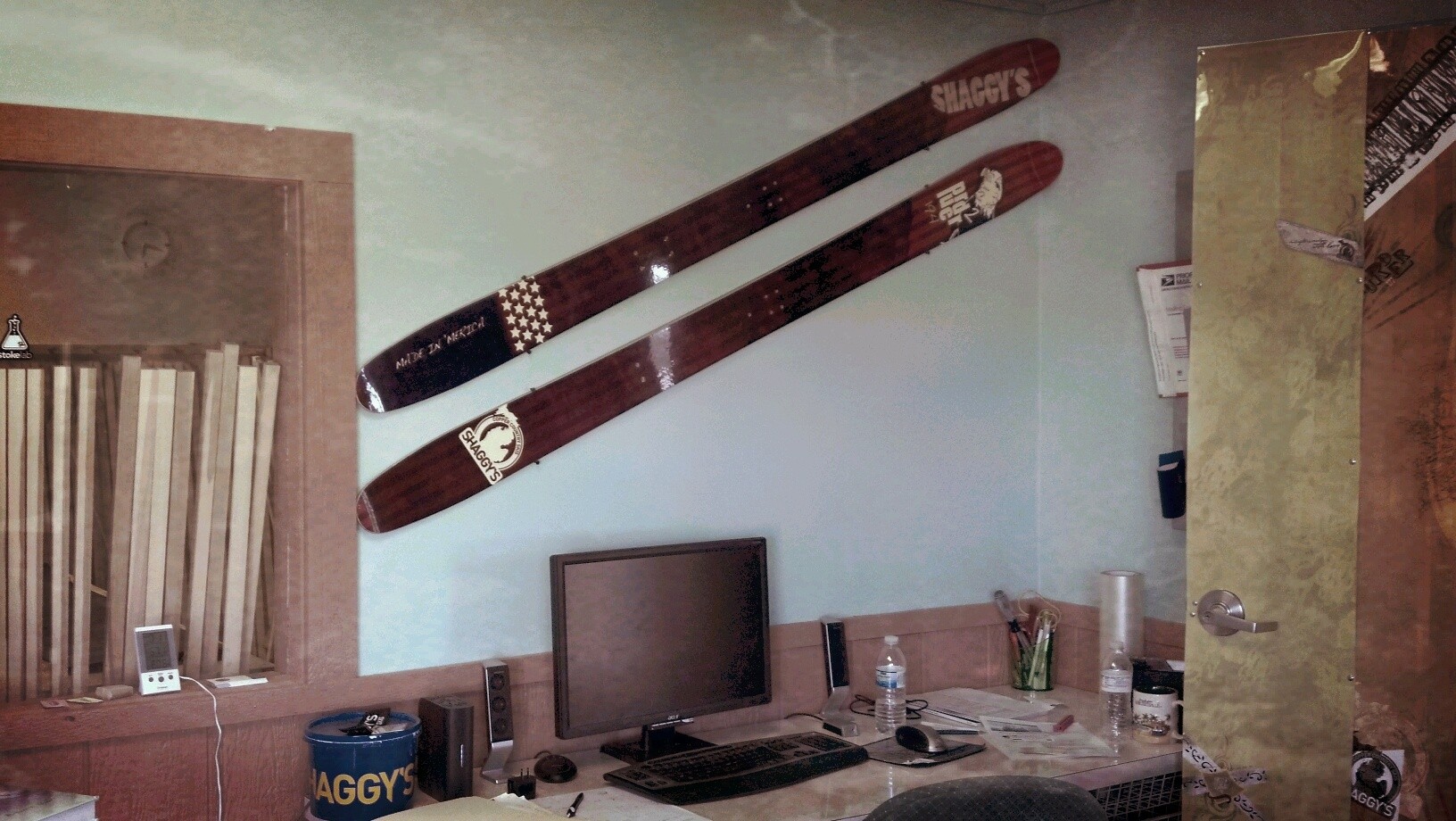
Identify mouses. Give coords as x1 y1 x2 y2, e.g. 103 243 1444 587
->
893 723 949 755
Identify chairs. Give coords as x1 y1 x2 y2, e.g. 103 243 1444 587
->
862 776 1112 821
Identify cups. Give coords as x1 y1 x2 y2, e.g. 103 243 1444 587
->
1133 686 1183 746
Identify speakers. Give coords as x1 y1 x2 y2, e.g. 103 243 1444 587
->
479 658 516 788
820 615 856 721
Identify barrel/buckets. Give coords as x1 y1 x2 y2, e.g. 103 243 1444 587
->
304 710 423 821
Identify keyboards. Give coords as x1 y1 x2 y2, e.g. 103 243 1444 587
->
601 728 869 807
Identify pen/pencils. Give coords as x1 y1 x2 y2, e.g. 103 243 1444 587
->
937 729 987 734
567 793 584 818
994 589 1052 691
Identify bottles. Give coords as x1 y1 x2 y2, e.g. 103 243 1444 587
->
875 635 906 736
1100 642 1135 740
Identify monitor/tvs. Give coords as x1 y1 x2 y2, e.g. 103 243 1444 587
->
548 536 774 766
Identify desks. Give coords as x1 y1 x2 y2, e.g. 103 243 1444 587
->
306 682 1184 821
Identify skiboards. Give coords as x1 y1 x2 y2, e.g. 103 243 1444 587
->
355 33 1067 534
